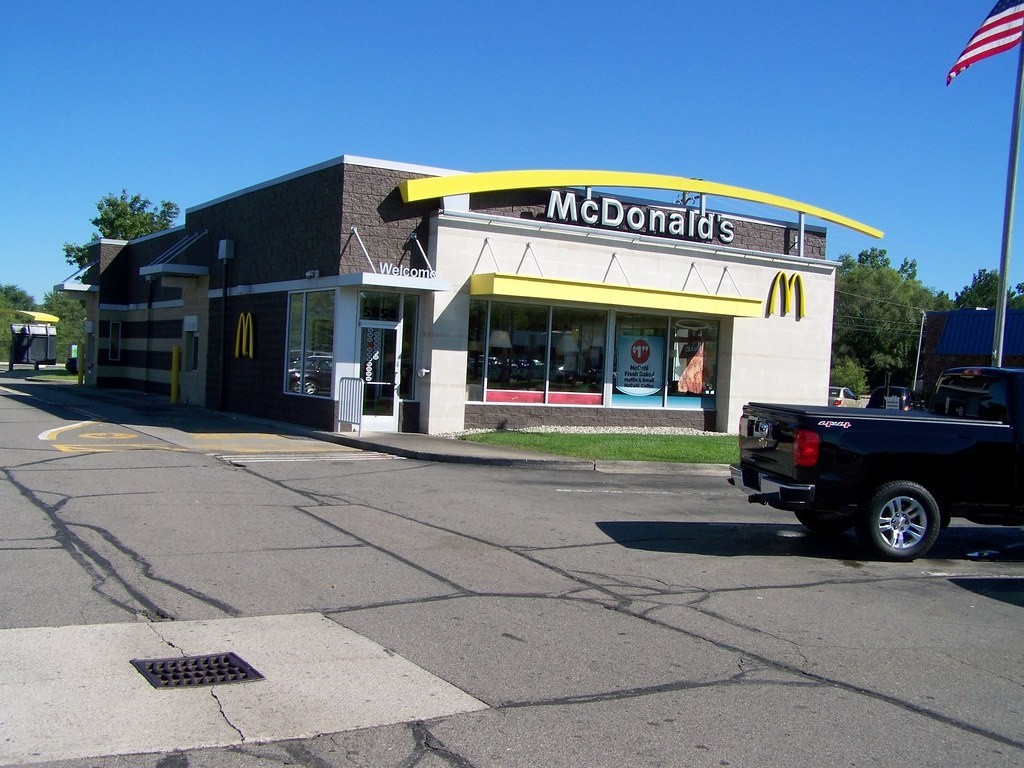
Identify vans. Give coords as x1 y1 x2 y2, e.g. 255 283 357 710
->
865 384 925 412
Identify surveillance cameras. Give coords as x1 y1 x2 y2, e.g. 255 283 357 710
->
306 271 314 279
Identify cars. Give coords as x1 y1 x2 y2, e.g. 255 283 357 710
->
829 386 863 409
469 354 603 385
289 349 334 397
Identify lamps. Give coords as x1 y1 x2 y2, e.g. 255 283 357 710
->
489 304 515 349
554 309 580 352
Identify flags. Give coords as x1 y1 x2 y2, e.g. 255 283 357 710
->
945 0 1024 88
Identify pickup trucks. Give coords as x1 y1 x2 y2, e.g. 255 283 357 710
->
723 367 1024 563
